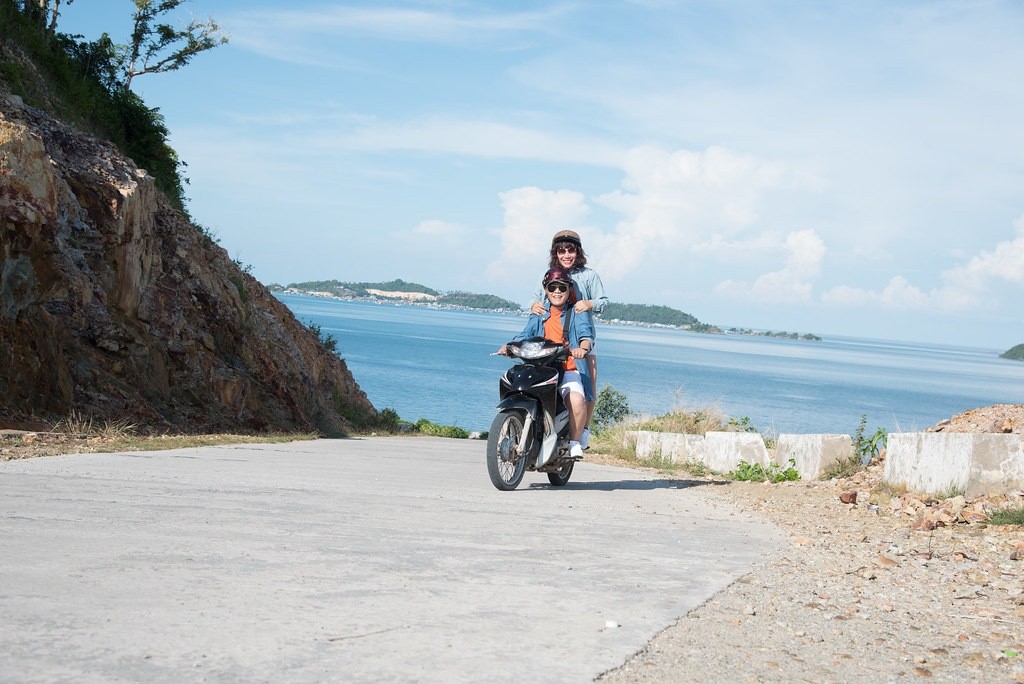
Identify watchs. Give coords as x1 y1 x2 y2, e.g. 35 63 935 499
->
582 348 588 358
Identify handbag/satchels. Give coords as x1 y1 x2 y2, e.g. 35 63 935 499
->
547 361 565 388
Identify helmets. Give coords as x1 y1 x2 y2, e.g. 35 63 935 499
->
553 230 580 244
542 268 573 287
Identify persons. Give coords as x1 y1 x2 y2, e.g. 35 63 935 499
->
498 267 592 461
528 231 607 452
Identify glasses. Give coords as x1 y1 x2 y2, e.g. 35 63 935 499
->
548 285 568 293
557 248 576 254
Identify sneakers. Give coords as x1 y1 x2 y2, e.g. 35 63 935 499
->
581 428 591 450
568 441 584 459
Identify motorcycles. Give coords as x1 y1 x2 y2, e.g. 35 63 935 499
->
486 336 577 491
